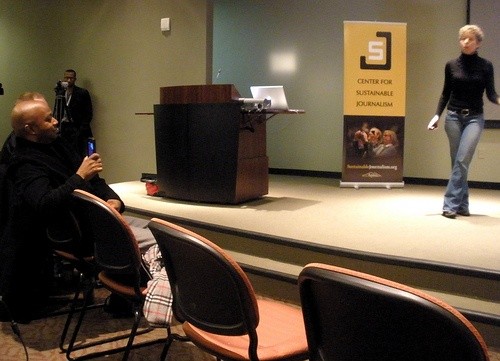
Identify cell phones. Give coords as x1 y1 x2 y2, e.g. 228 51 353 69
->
87 138 96 157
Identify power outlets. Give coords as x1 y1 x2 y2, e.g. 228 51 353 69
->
160 18 170 32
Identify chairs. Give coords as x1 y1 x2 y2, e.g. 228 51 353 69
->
49 184 159 353
149 218 308 361
67 187 152 361
296 263 499 360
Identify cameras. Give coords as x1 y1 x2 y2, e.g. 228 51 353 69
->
55 80 69 96
0 83 4 95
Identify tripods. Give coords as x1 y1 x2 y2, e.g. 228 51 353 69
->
52 95 74 131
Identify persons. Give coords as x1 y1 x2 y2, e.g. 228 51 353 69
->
0 91 127 263
53 69 94 178
428 24 500 220
347 120 401 164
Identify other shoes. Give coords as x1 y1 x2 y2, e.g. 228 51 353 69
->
443 210 457 218
108 294 147 319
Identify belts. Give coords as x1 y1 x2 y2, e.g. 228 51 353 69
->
447 105 483 117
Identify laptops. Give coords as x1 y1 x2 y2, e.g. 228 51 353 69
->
250 85 304 112
